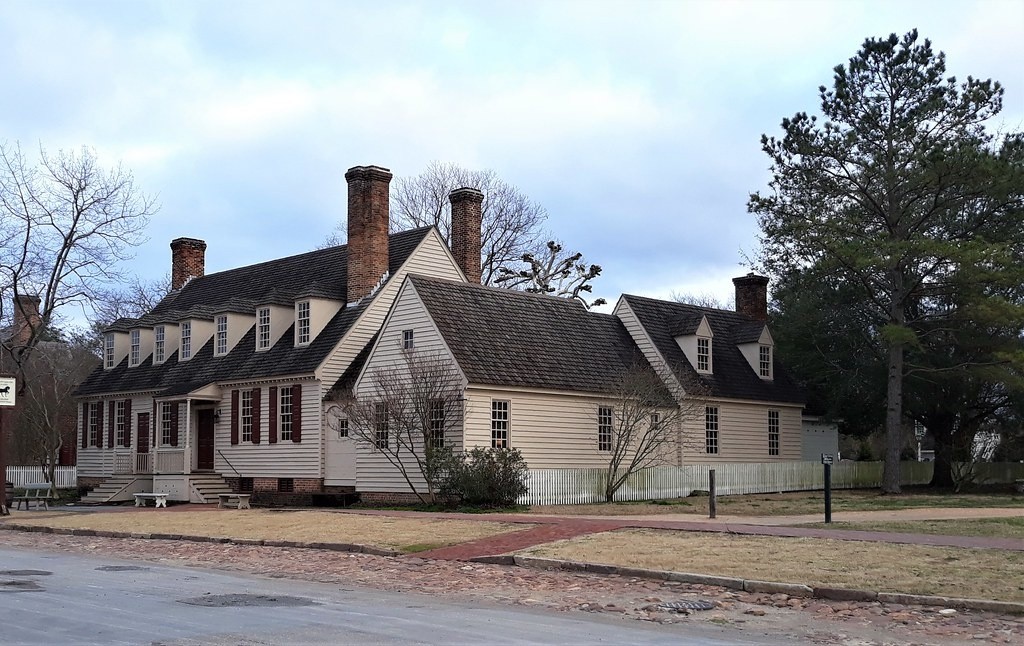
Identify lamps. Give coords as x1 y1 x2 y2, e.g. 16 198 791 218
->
213 409 221 424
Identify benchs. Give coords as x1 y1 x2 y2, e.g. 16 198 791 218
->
134 493 169 508
217 494 251 509
13 483 53 511
